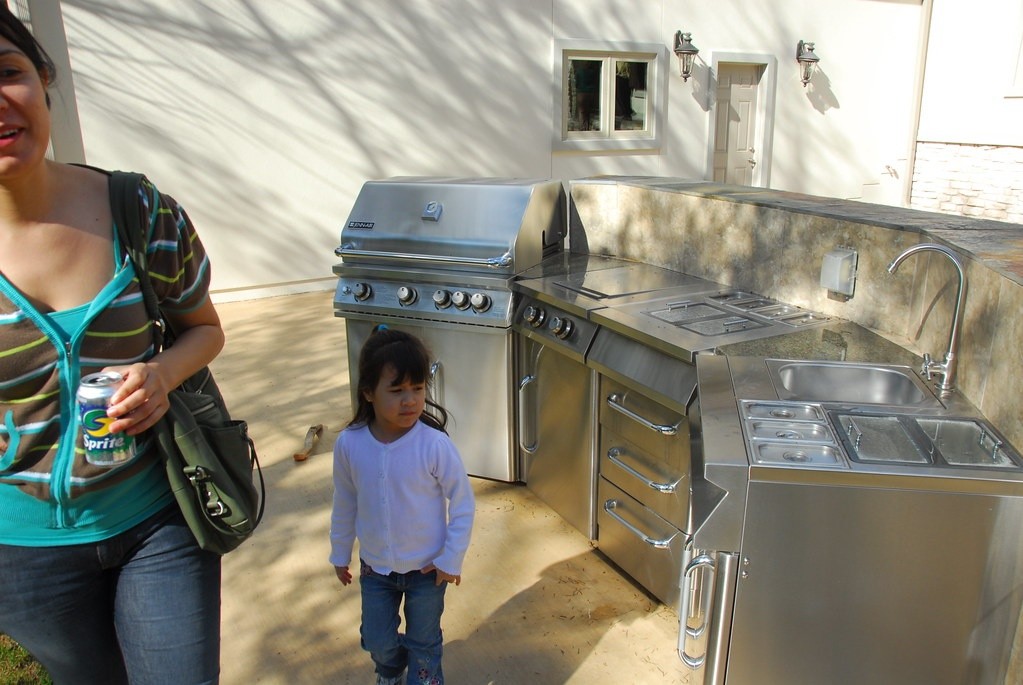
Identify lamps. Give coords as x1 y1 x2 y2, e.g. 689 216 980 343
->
672 29 700 83
795 39 821 87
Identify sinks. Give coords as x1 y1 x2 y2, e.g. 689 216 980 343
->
765 359 946 412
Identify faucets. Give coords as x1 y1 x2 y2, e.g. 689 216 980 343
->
888 242 968 390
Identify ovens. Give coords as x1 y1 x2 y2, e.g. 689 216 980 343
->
512 296 599 539
332 261 519 484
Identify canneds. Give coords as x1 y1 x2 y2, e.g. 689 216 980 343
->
76 371 138 468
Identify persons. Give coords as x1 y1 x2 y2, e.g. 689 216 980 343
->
0 0 226 684
329 321 475 685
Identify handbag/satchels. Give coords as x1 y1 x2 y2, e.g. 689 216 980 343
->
108 170 267 554
